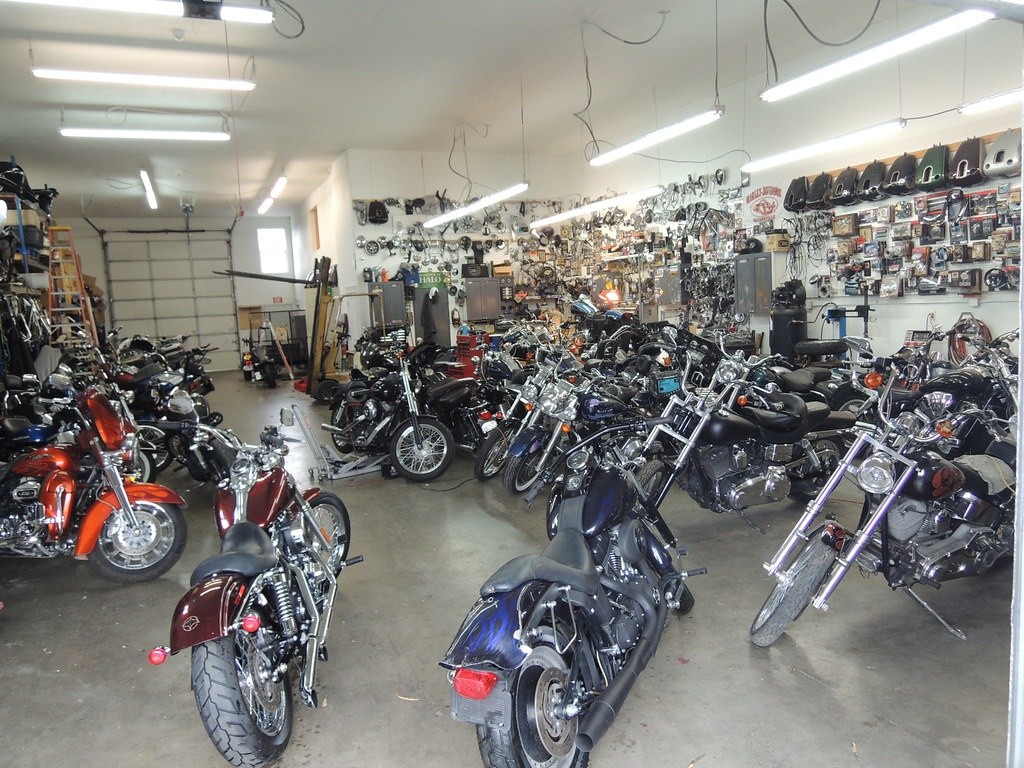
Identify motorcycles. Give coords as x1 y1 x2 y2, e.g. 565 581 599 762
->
156 396 365 768
1 279 1019 649
435 416 708 768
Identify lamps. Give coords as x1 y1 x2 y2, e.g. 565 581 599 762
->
53 104 232 143
26 37 257 94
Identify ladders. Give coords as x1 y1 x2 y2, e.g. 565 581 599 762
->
48 226 100 375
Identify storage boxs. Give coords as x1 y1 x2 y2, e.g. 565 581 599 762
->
766 229 787 235
766 234 789 252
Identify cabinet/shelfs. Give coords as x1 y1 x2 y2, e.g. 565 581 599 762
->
653 263 692 305
368 281 406 326
465 278 500 320
413 287 451 353
735 251 786 316
